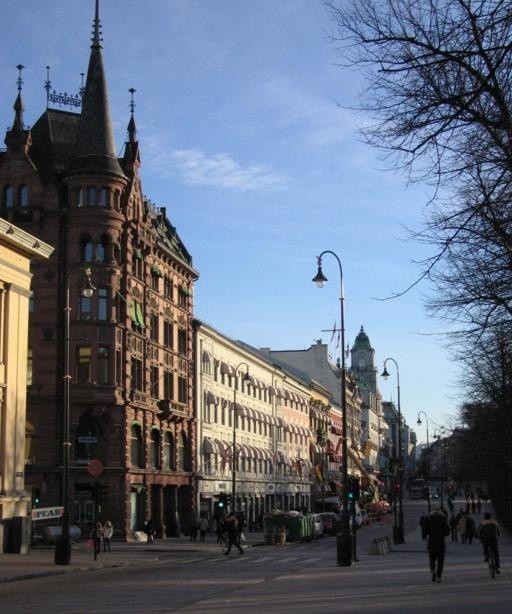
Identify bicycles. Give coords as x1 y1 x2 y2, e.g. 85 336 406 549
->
487 545 496 580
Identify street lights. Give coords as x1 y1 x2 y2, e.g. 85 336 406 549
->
51 263 102 568
229 362 254 513
380 357 406 546
416 411 433 514
311 248 357 568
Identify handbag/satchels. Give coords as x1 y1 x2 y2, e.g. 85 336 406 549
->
428 534 446 552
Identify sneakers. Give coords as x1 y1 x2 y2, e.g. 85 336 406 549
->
432 572 441 583
496 567 500 574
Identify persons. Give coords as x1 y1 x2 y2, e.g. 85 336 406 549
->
419 485 489 545
144 508 248 555
475 511 503 574
88 520 113 553
421 499 450 582
